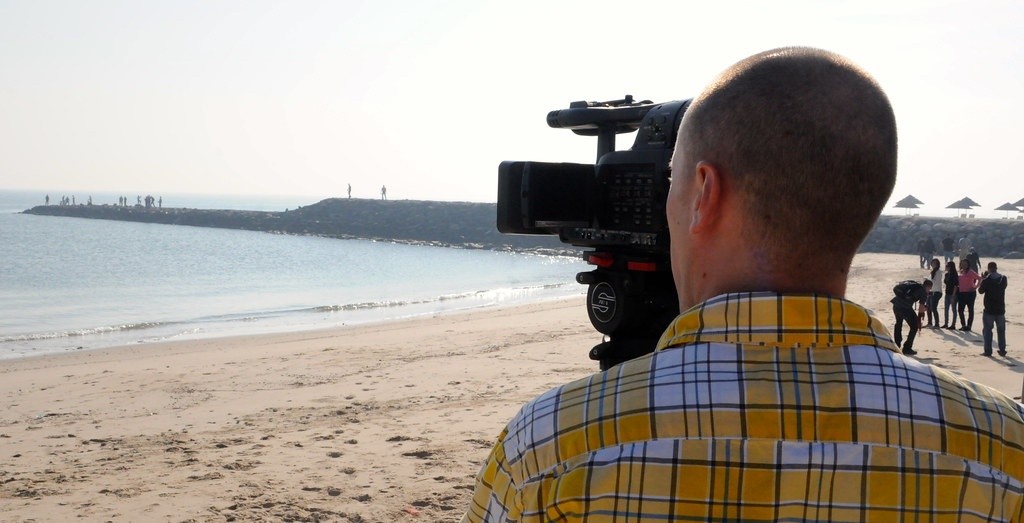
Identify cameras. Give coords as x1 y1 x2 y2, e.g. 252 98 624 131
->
984 271 989 275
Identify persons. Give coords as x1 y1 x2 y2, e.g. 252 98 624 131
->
119 196 127 206
890 279 933 354
347 184 352 198
46 195 49 203
382 185 387 200
461 47 1024 523
62 195 75 205
88 195 92 204
137 195 162 207
918 233 980 332
979 262 1007 357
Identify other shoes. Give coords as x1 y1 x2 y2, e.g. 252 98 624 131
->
932 324 939 328
926 324 931 326
998 350 1006 356
959 326 970 330
902 348 918 355
981 352 991 356
941 325 948 328
947 326 955 329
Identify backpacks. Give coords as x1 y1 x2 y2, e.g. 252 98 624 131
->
893 280 924 303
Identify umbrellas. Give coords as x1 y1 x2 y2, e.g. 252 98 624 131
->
994 197 1024 218
893 195 923 215
945 197 981 217
